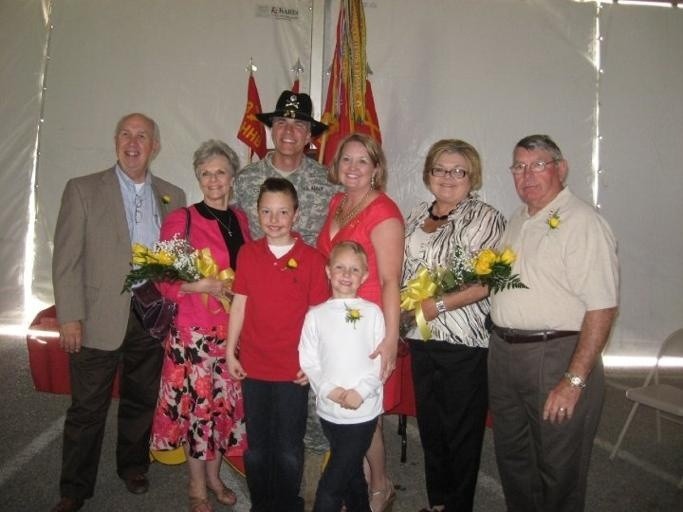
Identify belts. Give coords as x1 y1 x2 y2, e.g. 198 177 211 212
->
485 314 580 343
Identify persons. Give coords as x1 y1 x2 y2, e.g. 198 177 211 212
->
53 113 186 512
225 179 332 512
486 134 621 512
401 140 508 512
155 140 253 512
230 90 345 245
298 240 386 512
315 131 405 512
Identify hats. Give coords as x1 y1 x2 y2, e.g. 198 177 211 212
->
255 89 329 137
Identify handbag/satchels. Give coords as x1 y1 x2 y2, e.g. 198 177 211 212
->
131 207 191 341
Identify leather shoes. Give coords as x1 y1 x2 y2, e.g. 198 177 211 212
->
49 496 84 512
125 474 149 494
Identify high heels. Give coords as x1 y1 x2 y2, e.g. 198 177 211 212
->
369 477 397 512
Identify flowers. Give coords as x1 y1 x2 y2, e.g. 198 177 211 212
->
472 245 530 296
161 193 173 206
546 206 561 231
345 306 362 325
284 257 299 270
130 231 201 279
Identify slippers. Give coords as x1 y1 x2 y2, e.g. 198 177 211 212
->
208 483 237 507
189 495 212 512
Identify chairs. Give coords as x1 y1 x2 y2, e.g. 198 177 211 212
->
608 328 683 464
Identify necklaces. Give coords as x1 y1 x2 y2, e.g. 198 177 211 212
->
427 199 464 221
330 186 375 225
202 200 235 238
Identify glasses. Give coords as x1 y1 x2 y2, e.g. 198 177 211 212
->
431 168 469 179
509 159 555 175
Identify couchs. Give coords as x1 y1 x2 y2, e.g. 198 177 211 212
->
25 304 123 399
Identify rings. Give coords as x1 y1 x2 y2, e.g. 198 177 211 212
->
559 407 566 412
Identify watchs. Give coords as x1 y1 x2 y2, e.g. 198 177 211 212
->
562 369 588 392
434 294 447 315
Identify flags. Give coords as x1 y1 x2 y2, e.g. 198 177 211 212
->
236 74 267 160
313 0 381 164
